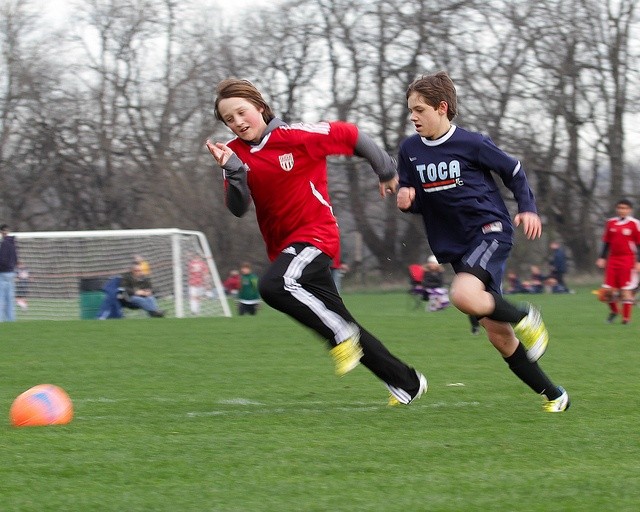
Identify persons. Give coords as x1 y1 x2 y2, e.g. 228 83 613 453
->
520 264 544 294
117 265 169 317
596 198 640 324
223 268 242 296
132 252 150 276
544 276 569 294
206 78 428 407
540 239 568 278
14 256 31 313
419 254 450 312
502 269 523 294
397 72 570 413
330 244 350 297
0 223 19 322
237 261 261 316
185 252 210 315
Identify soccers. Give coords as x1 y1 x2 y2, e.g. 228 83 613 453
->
10 384 74 426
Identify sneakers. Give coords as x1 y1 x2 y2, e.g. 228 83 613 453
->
513 302 549 364
386 370 428 410
541 384 571 413
329 321 365 379
